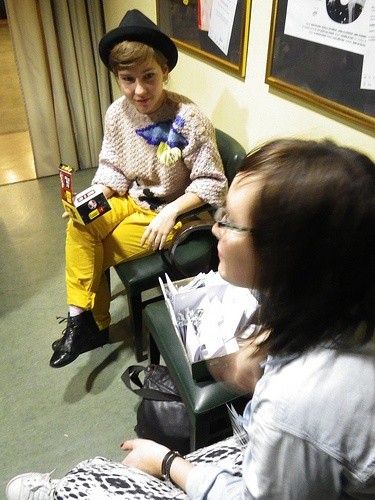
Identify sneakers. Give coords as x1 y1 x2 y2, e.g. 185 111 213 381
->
5 468 61 500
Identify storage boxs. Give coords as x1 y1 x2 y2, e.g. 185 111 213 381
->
158 272 221 383
57 163 112 226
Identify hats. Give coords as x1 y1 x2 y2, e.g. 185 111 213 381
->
98 8 178 74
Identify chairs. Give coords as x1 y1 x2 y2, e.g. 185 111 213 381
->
141 301 252 454
106 128 246 363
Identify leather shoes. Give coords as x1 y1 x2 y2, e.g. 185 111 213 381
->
52 327 109 350
50 310 101 368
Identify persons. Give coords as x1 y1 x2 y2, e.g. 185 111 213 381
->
3 138 375 500
50 9 228 368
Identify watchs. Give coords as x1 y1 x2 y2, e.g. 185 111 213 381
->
160 450 184 483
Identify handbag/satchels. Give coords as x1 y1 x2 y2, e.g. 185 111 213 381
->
120 363 233 456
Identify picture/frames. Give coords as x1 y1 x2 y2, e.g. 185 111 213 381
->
156 0 252 78
265 0 375 130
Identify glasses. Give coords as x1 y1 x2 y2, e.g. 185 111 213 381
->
213 207 254 237
